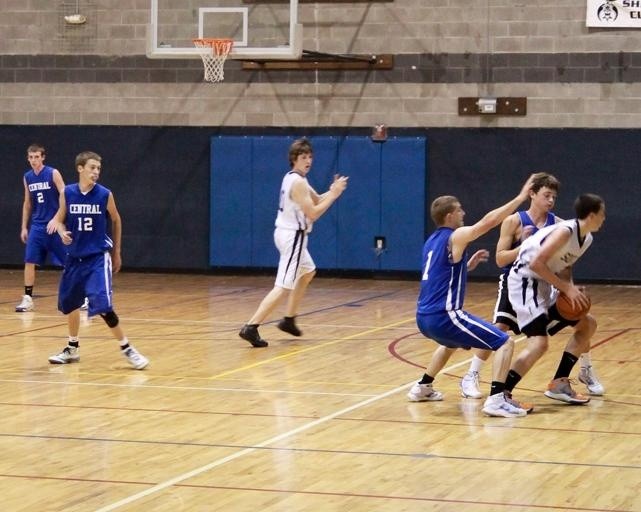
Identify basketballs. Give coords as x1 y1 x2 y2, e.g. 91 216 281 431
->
555 290 592 321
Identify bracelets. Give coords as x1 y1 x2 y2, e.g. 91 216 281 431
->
552 278 562 290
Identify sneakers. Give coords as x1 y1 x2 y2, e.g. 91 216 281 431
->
459 373 483 399
482 391 527 418
278 316 301 336
15 295 34 312
407 380 444 402
238 323 268 348
121 345 149 371
543 376 590 403
504 394 534 414
578 364 604 395
48 345 80 365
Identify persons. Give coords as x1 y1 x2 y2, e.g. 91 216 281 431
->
236 138 351 349
503 189 606 408
404 171 543 419
47 151 151 373
13 144 89 314
458 172 606 400
